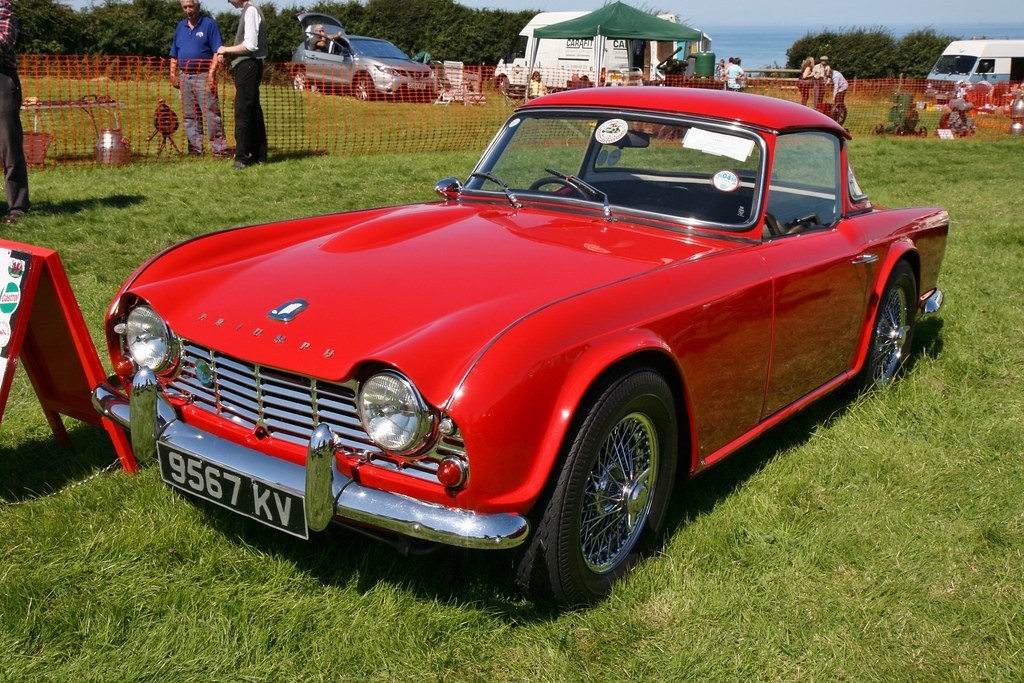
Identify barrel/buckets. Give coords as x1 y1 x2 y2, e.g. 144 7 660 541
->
694 50 715 78
95 125 128 169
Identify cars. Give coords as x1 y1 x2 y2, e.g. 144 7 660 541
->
88 81 951 626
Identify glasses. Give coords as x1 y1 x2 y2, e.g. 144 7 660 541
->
316 30 324 33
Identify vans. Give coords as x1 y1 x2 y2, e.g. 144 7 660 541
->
924 38 1024 106
490 8 678 96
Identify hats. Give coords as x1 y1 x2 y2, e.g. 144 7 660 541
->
820 56 828 60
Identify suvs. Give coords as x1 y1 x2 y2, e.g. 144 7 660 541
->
287 11 434 101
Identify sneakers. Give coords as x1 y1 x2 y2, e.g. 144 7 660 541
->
231 161 246 171
3 212 21 225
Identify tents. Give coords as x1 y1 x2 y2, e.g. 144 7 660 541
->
523 0 706 108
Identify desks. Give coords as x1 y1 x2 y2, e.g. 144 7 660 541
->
20 97 123 136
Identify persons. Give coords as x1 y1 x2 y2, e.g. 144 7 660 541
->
715 59 726 82
168 0 232 159
824 65 849 111
726 57 745 92
797 56 814 106
307 24 342 54
529 71 549 98
951 56 995 73
567 73 594 89
0 0 32 226
811 55 831 110
722 57 735 90
216 0 269 170
